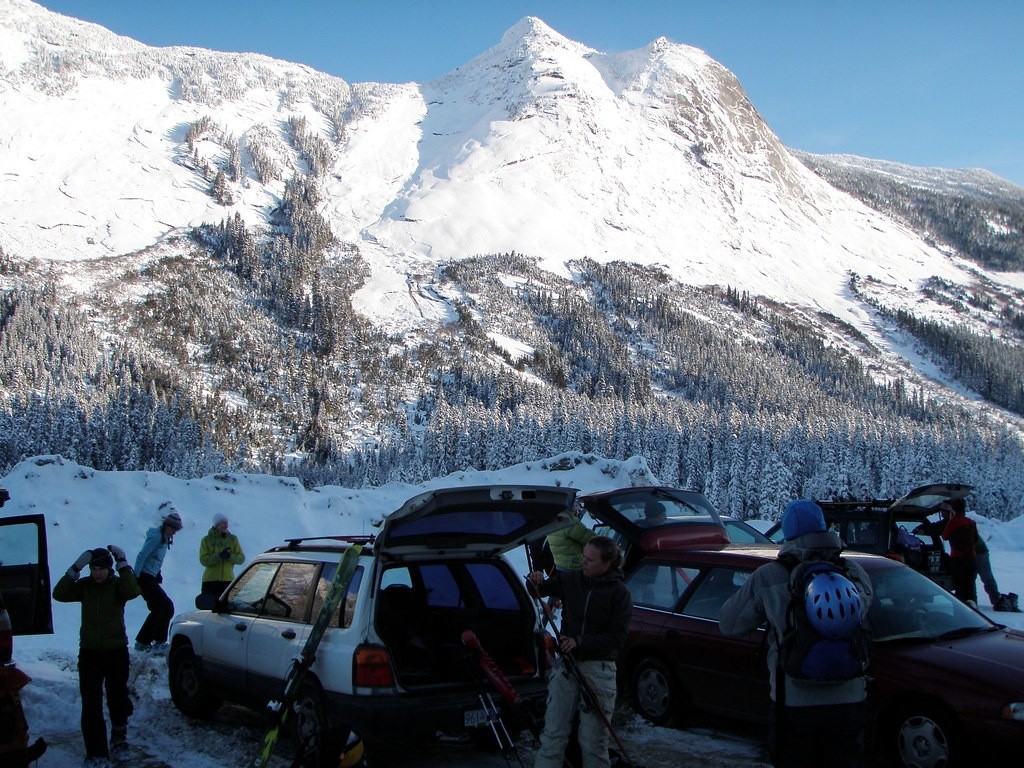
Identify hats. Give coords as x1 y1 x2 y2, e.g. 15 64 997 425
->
940 503 952 512
89 548 113 569
212 513 226 527
943 496 965 514
162 513 182 545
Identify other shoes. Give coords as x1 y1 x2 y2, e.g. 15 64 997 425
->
110 737 129 753
994 592 1019 612
84 756 110 767
135 641 169 652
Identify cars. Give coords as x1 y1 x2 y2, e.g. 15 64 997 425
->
592 513 782 608
579 487 1024 767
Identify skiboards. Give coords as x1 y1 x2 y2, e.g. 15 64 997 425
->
250 542 362 768
461 629 542 738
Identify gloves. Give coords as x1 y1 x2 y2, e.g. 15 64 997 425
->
67 550 92 581
156 571 162 583
28 736 47 759
107 545 127 571
219 551 230 559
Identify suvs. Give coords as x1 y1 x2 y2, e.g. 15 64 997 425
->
0 492 62 767
163 484 584 750
745 483 976 604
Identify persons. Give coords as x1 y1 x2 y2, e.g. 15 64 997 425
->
52 545 143 768
199 513 246 599
720 499 873 768
526 535 634 768
546 490 623 655
617 502 667 604
133 513 183 653
0 666 48 768
941 497 979 608
912 503 1001 606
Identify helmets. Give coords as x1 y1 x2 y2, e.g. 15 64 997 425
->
802 561 861 639
782 499 826 538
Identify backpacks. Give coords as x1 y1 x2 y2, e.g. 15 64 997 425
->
777 551 868 685
